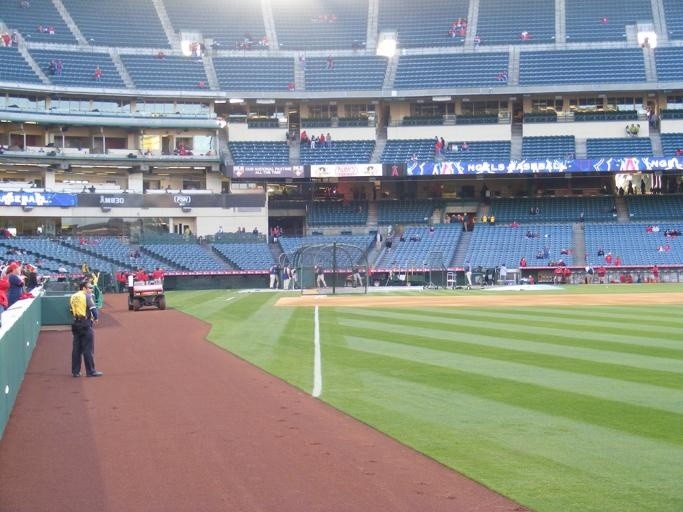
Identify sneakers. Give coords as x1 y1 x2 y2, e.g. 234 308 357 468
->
87 372 103 377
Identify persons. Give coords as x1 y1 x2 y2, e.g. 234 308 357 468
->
0 23 55 46
644 224 683 239
519 30 531 41
398 210 475 242
446 17 480 45
599 15 608 26
434 135 470 156
390 164 399 177
525 229 550 259
181 224 286 244
599 178 647 195
310 14 338 24
623 124 641 138
172 144 192 155
509 219 521 228
47 59 63 77
94 65 102 82
384 233 392 254
268 262 300 291
409 153 417 161
480 212 496 226
283 129 332 149
460 259 508 287
529 207 540 216
658 245 670 252
343 265 363 288
188 31 270 57
313 264 326 289
287 81 296 91
0 218 167 378
18 1 31 10
496 71 507 82
519 248 659 283
326 54 334 70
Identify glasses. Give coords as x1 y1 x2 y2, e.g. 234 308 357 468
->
87 287 92 289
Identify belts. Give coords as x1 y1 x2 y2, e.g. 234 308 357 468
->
73 318 80 321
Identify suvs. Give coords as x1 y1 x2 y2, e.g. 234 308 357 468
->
124 275 166 311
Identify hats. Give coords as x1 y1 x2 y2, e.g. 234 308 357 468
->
9 263 18 270
27 264 32 269
31 266 37 269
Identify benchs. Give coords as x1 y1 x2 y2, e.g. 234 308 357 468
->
0 0 683 285
